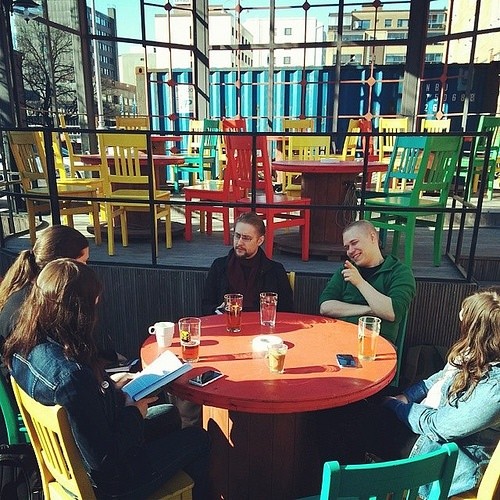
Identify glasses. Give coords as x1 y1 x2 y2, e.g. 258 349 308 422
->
231 232 254 242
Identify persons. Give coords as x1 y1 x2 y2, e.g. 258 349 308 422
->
316 284 500 500
0 259 211 500
318 219 416 398
199 211 295 319
0 227 169 411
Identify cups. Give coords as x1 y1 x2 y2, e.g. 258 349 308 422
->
224 294 243 332
149 322 174 348
260 292 278 327
178 317 201 362
267 344 288 373
358 316 381 362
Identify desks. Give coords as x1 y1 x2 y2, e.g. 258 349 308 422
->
272 160 389 258
80 154 185 240
151 135 183 189
140 312 398 500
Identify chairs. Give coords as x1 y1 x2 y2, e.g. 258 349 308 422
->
173 119 330 261
341 115 500 268
10 374 193 500
452 439 500 500
6 115 172 256
0 373 31 445
296 441 458 500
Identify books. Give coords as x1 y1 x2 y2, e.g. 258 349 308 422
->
120 350 193 403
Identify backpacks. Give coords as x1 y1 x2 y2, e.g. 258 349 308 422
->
0 442 44 500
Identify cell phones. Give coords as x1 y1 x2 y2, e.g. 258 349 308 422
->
188 369 225 387
334 353 358 370
